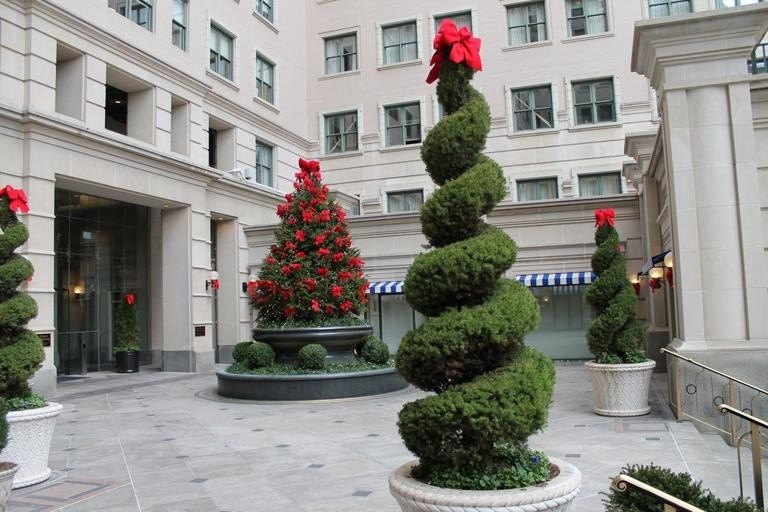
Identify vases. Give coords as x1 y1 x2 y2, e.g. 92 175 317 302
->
253 323 375 365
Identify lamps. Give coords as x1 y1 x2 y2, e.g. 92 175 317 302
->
630 272 641 294
205 270 218 291
648 267 665 292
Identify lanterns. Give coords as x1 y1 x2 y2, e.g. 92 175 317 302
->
246 273 258 296
630 275 640 296
206 271 219 289
648 267 664 295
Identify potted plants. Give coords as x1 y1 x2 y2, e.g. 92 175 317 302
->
217 341 407 399
0 397 20 512
584 207 657 418
0 184 64 493
112 287 143 374
389 18 582 512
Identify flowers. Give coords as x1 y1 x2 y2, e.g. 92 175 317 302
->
240 159 370 314
